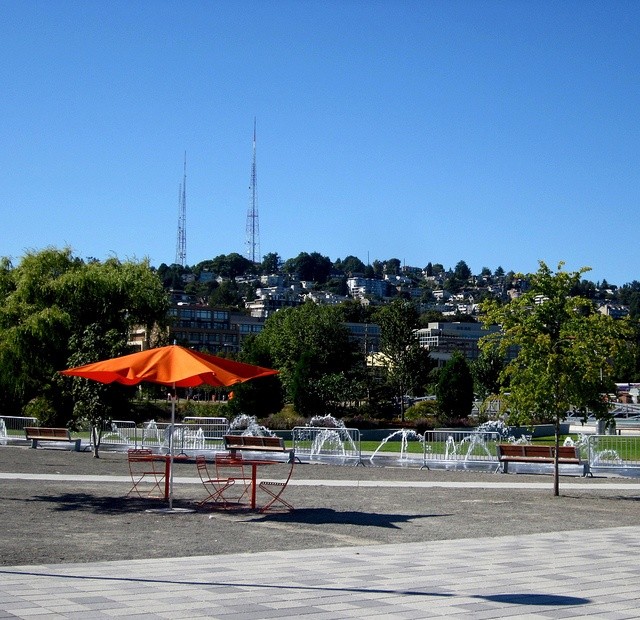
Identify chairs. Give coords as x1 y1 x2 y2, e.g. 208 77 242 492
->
124 444 177 506
194 446 236 514
260 446 296 515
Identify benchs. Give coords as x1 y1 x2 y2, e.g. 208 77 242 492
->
223 434 295 465
497 445 591 477
23 428 83 453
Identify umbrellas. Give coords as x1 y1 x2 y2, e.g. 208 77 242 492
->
56 339 283 510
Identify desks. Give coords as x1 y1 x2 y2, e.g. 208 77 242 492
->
145 454 286 510
